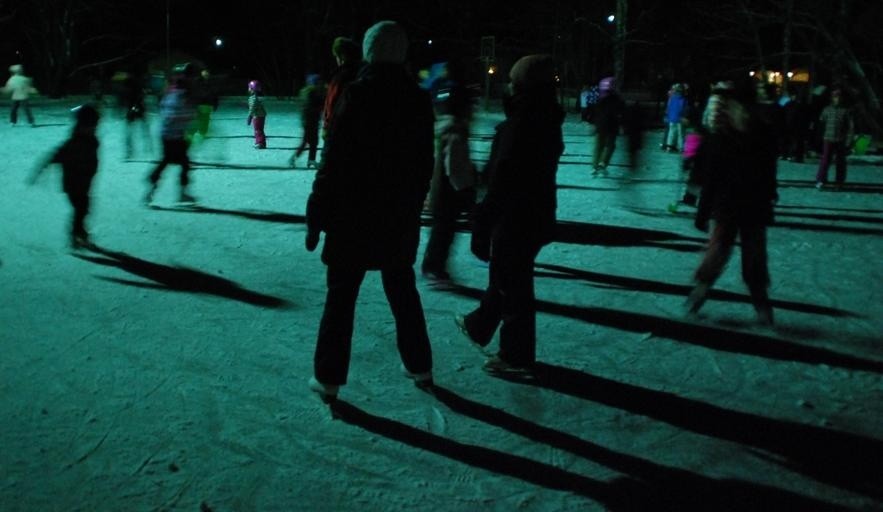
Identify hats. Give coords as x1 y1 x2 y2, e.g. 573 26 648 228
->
510 54 553 92
363 17 408 64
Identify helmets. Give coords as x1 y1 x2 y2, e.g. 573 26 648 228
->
249 79 262 92
598 77 619 92
307 73 323 85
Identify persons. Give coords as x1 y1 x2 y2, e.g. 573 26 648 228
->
683 81 778 327
6 64 36 127
187 70 219 139
28 106 99 248
454 54 565 383
665 83 687 154
305 21 435 402
322 37 366 149
421 76 479 282
816 90 854 192
143 63 196 203
246 80 268 149
115 79 152 160
783 90 805 164
583 76 627 175
288 74 325 168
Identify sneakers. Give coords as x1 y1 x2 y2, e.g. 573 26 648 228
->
307 159 320 166
178 194 197 203
400 362 433 387
307 375 339 401
483 356 535 373
455 315 485 347
